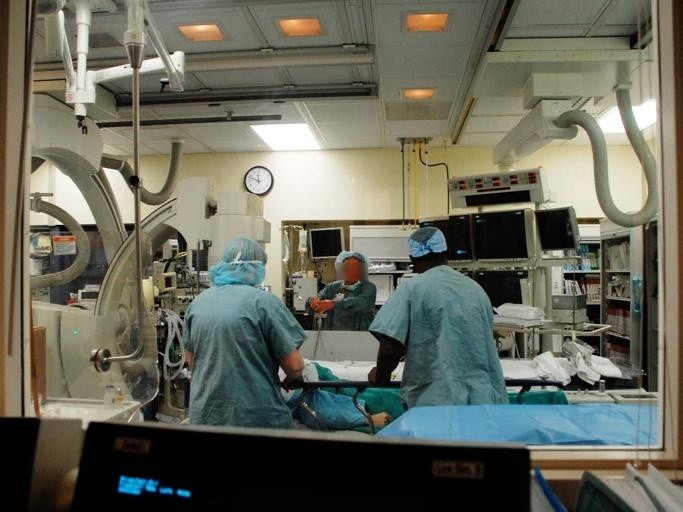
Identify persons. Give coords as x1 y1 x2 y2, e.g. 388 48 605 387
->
280 387 391 430
367 226 510 411
306 250 378 331
179 235 308 429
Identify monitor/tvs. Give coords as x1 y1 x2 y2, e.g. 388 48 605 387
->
309 227 345 260
418 212 472 262
66 421 531 512
472 207 535 262
534 206 580 251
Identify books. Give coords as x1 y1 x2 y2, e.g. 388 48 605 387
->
530 461 683 511
561 244 630 365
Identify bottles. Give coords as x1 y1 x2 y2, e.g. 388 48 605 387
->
605 308 629 362
564 245 599 271
580 280 600 295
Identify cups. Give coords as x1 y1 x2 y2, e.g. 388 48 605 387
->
632 278 641 314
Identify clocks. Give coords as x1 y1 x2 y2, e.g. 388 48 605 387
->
245 166 273 196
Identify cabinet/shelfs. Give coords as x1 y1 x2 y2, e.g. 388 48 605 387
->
599 211 644 389
561 240 600 355
